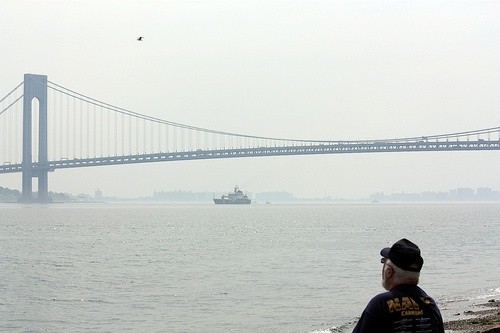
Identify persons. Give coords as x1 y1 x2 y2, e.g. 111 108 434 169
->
351 238 444 333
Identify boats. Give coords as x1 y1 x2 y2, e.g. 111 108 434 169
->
212 185 251 203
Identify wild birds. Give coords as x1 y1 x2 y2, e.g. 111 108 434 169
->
136 36 144 41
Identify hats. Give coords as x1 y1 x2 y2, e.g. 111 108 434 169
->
380 238 423 272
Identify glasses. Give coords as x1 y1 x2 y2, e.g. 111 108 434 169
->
381 258 395 273
